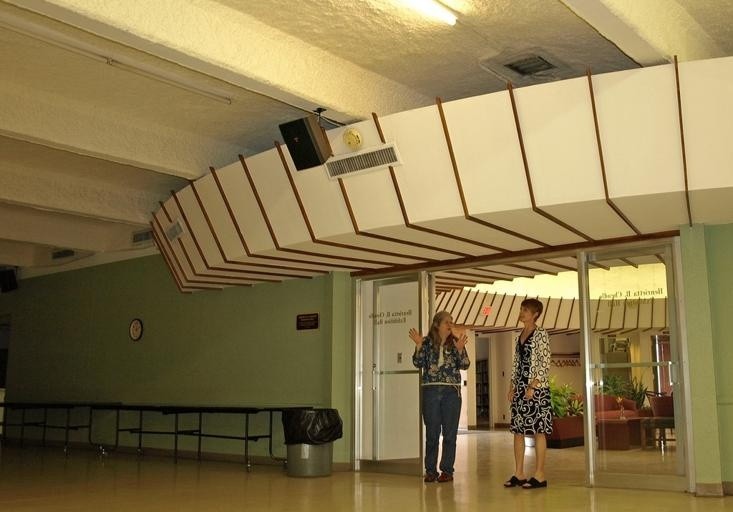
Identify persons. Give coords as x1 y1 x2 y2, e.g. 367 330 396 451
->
502 296 552 490
407 309 471 483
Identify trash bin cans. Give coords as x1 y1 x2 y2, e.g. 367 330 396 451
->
282 408 338 478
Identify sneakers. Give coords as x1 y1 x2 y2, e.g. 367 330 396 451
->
425 473 453 482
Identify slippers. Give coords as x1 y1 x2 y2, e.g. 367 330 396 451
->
504 476 546 488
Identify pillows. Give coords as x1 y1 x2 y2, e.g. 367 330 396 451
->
646 391 673 417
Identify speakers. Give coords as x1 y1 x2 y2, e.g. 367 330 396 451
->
278 115 332 172
0 270 18 294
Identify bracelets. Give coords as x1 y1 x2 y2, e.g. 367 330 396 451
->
527 386 535 391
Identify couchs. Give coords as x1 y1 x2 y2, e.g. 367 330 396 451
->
545 392 638 448
